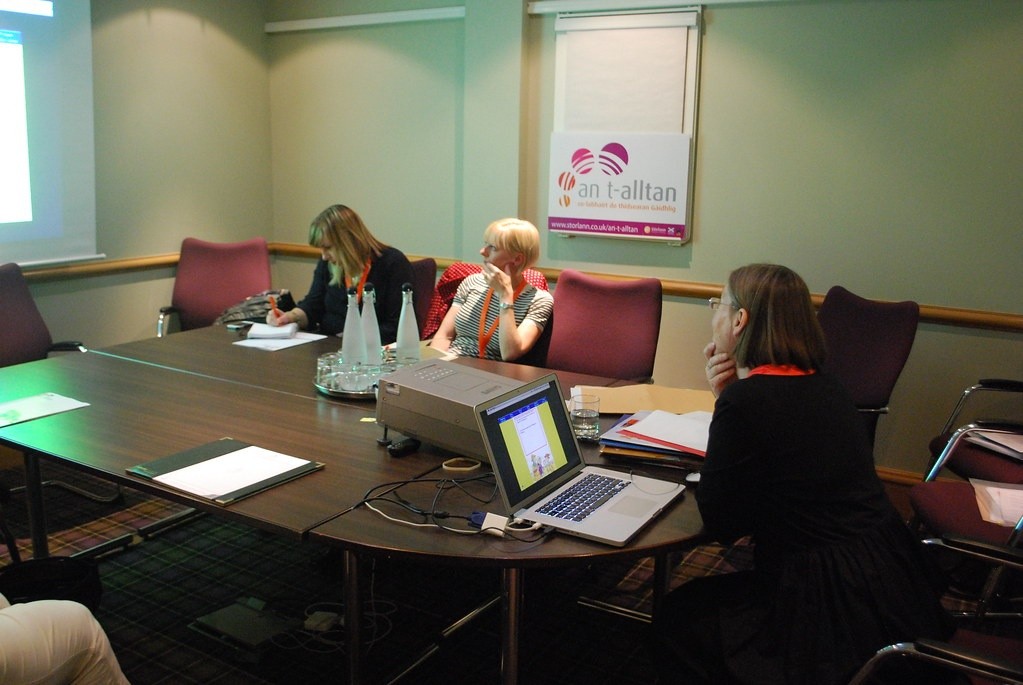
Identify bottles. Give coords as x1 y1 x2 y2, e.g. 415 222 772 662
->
395 282 422 362
359 283 383 363
341 286 368 365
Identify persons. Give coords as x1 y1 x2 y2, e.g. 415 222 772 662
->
0 591 130 684
649 264 958 684
427 218 555 367
265 204 415 345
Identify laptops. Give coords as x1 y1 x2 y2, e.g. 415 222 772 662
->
187 602 296 656
479 372 685 548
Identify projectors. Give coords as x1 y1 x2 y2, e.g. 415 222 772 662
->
374 359 528 464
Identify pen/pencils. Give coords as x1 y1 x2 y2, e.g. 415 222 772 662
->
269 296 283 326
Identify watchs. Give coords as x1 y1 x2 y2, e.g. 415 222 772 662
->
498 303 515 310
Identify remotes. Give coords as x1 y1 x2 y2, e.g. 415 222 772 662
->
387 439 421 457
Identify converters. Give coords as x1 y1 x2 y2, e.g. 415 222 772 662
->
304 611 337 631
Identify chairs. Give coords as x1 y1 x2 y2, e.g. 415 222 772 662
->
444 263 547 323
0 263 124 503
156 238 273 337
905 379 1023 481
847 535 1023 685
816 285 919 452
410 258 436 338
545 272 662 384
904 481 1023 632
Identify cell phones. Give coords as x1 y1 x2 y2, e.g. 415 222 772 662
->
227 321 255 329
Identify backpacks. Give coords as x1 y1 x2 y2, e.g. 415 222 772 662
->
0 556 102 615
213 289 296 326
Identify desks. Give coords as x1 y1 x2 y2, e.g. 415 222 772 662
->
0 320 706 685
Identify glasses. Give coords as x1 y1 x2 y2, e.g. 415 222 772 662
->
709 297 739 311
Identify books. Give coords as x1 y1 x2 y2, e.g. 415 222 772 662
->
597 409 713 470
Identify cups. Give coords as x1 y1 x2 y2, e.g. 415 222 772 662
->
569 394 600 442
316 346 420 392
